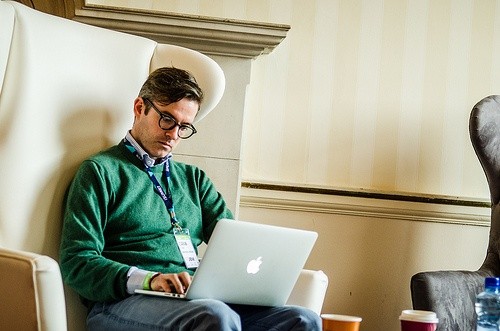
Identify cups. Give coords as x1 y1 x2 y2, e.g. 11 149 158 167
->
320 314 362 331
398 310 440 331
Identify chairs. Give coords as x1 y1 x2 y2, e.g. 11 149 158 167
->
0 0 329 331
410 95 500 331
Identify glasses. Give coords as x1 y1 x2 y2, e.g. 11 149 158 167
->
145 99 197 139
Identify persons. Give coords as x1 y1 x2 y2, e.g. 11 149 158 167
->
59 67 323 331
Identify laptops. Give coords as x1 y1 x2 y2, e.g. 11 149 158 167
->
135 219 318 307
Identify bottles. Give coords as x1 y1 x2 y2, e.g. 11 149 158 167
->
475 276 500 331
